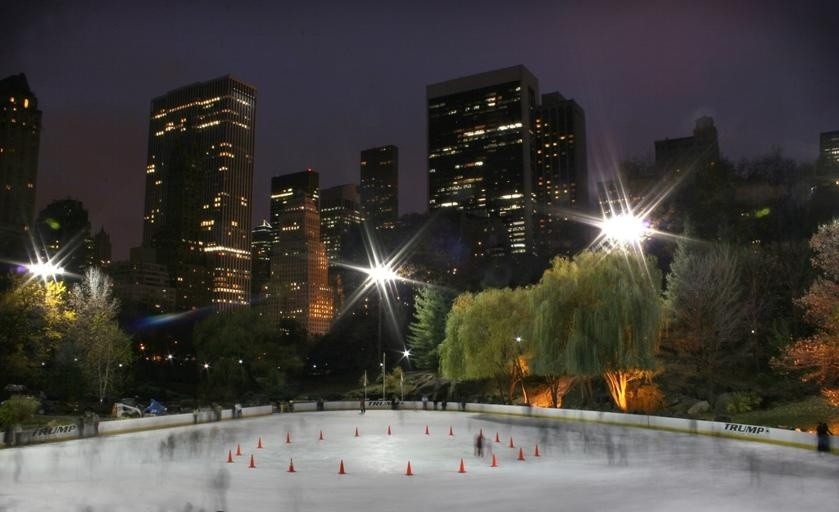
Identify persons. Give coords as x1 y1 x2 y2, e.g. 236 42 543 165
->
207 404 211 423
193 404 198 425
360 397 365 413
817 420 834 453
215 402 221 420
320 398 324 410
288 399 294 412
232 402 236 418
442 397 446 409
317 398 319 411
461 397 465 411
392 397 396 409
477 433 483 457
421 393 428 409
276 399 280 412
433 396 438 410
234 403 243 418
281 399 286 412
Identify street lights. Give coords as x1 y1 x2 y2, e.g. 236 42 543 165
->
600 213 645 246
28 262 65 281
370 264 397 401
402 350 410 358
